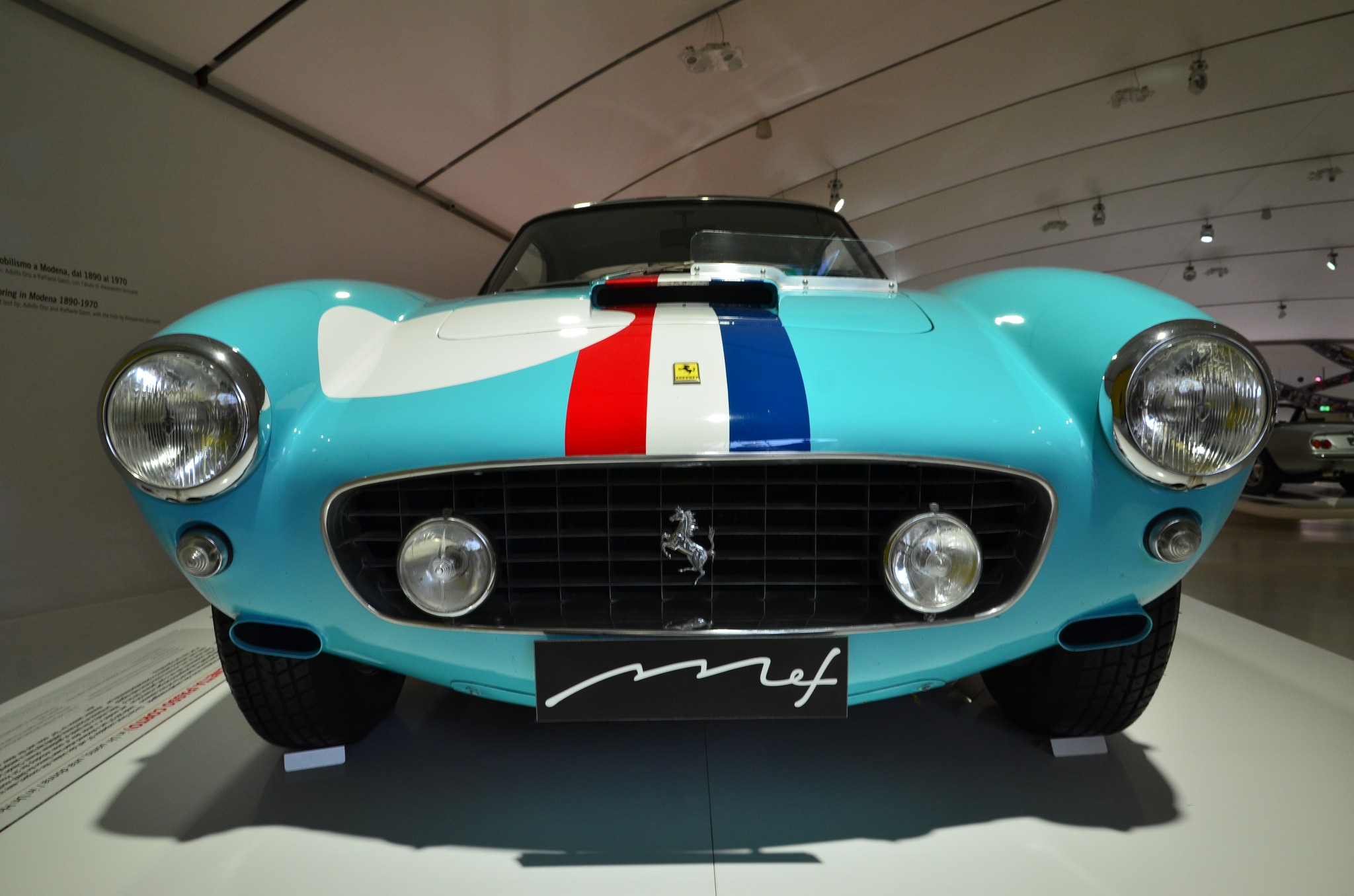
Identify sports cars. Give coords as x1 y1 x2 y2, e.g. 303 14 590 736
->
1244 400 1354 497
95 196 1270 760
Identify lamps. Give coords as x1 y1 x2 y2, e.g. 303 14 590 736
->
827 168 845 212
1040 50 1345 318
680 43 749 72
755 117 773 139
